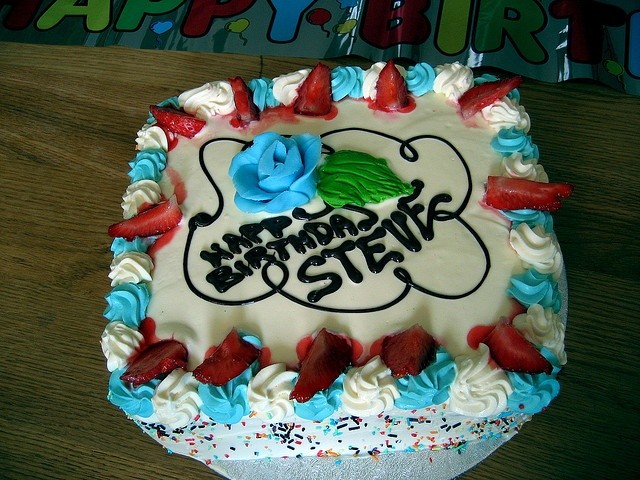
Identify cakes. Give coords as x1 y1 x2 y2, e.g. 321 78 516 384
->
101 62 572 479
